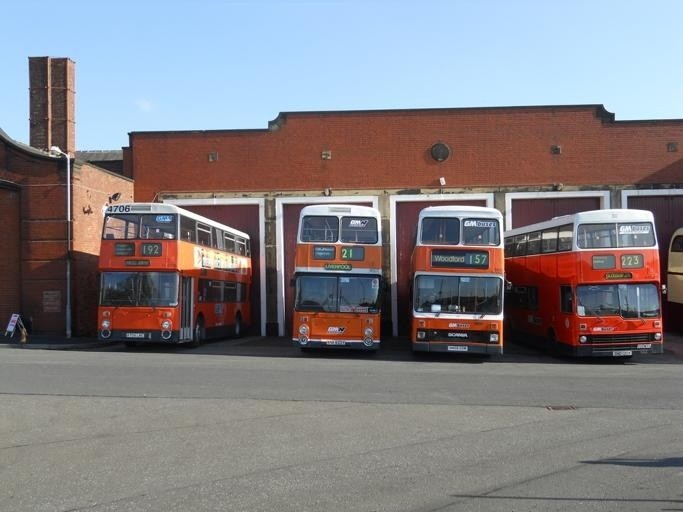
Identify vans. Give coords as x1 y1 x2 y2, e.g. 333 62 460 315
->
664 225 682 328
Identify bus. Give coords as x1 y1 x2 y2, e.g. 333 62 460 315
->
407 205 512 359
504 209 667 360
289 204 390 356
95 202 253 349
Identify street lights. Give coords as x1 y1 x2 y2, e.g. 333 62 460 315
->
50 146 72 339
